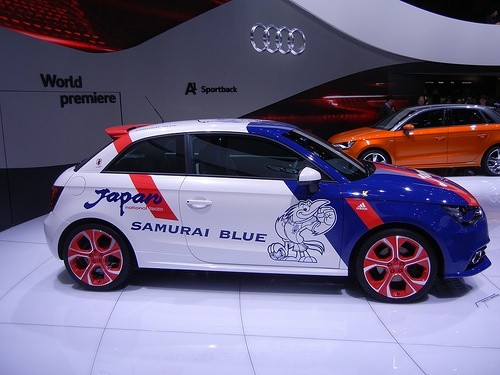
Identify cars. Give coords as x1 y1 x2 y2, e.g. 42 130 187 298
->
327 103 500 177
44 118 492 305
